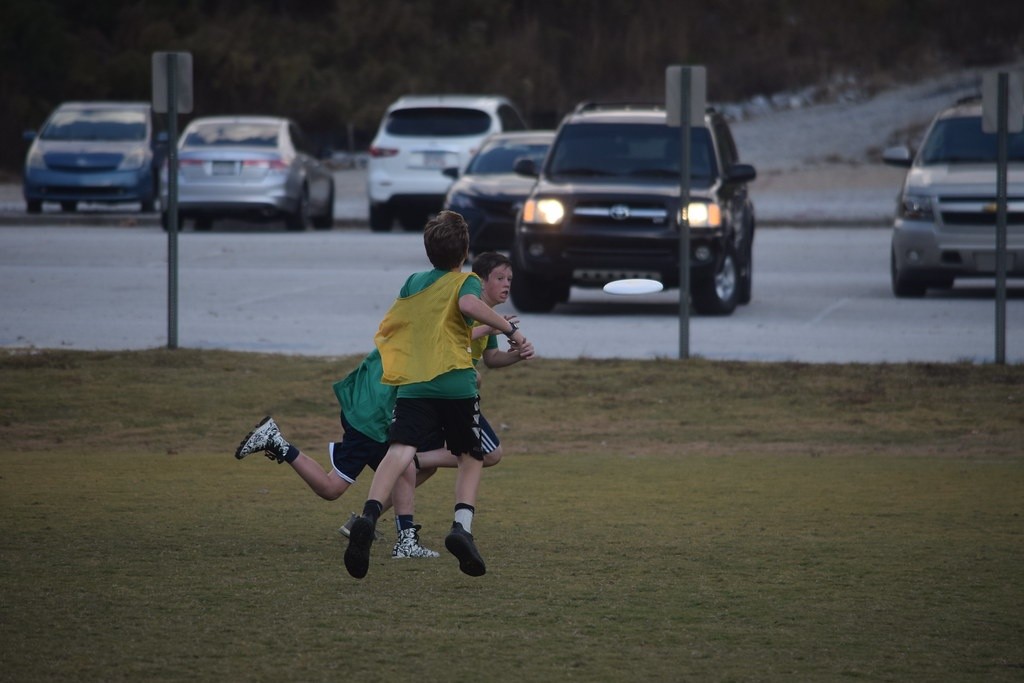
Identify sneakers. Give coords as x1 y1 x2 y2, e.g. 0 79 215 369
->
344 517 375 578
444 521 486 577
336 512 388 542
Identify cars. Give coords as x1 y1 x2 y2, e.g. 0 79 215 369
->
438 131 559 258
23 100 167 214
158 114 338 235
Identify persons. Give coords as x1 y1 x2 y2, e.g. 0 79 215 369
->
235 210 535 579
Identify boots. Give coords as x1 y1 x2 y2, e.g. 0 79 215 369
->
389 526 440 559
235 416 290 465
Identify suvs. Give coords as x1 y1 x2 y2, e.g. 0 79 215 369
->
366 98 531 235
503 95 761 323
879 95 1022 301
